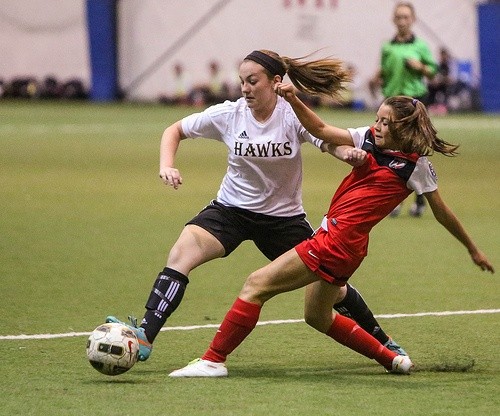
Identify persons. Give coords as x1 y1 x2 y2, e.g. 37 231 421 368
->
105 50 410 363
158 49 464 113
168 83 495 379
367 4 437 218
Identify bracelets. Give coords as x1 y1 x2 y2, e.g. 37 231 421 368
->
422 65 425 72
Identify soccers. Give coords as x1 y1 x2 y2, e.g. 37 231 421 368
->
86 322 139 376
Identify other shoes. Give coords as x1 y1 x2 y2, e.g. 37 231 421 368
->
390 203 402 215
411 203 425 215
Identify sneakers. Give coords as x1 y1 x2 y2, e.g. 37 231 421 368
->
385 355 414 374
168 358 228 377
105 315 154 361
385 337 407 357
